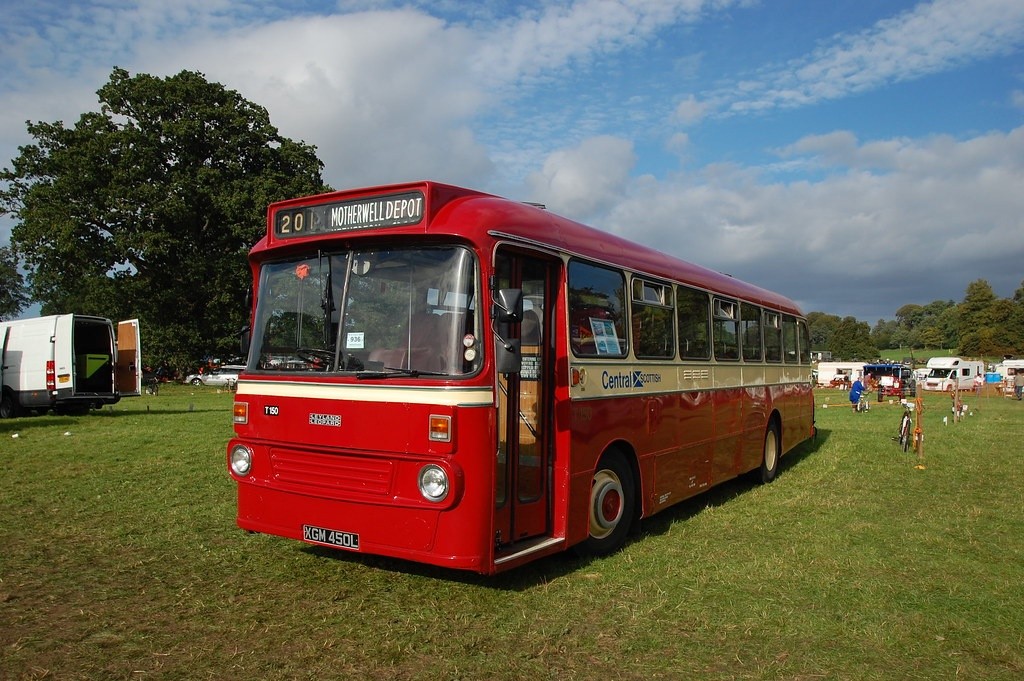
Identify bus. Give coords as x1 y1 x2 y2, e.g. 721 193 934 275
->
228 179 816 576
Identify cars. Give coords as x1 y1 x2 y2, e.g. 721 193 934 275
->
185 365 247 391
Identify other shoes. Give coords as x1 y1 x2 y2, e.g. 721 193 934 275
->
1019 397 1022 400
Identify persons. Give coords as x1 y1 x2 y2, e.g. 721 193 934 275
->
909 379 916 397
810 375 818 388
973 374 984 395
849 378 866 413
843 374 850 390
1013 370 1024 400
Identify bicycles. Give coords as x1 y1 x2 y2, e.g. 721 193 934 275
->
892 399 914 452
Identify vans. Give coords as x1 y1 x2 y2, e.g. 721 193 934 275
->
924 357 984 391
0 314 141 418
818 361 926 402
988 360 1024 395
